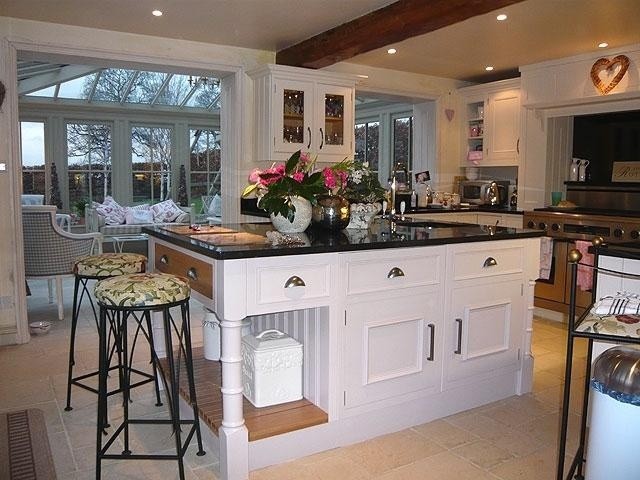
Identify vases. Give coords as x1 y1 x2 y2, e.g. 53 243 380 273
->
268 196 381 234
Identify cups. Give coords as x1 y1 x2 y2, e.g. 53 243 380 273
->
551 191 562 206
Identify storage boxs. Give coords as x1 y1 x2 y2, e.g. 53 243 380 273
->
241 329 305 409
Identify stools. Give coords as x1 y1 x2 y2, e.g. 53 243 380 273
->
64 254 206 480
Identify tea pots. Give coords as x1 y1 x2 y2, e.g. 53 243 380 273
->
569 157 590 182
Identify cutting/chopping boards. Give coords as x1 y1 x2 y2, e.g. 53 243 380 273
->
188 232 269 246
159 225 237 235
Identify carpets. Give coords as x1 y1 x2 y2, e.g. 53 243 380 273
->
1 410 57 479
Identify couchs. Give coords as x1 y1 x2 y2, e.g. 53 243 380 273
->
85 202 196 234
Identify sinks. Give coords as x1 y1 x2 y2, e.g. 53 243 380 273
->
393 219 480 230
408 207 471 212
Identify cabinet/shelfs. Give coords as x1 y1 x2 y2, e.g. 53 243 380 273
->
517 43 640 106
517 106 574 212
145 213 547 479
248 65 357 165
535 238 593 308
455 77 521 168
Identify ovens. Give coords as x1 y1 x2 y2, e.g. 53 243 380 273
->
533 240 567 304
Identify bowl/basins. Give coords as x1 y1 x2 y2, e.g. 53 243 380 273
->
466 168 479 181
30 320 51 334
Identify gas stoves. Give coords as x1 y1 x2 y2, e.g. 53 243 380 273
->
523 206 640 245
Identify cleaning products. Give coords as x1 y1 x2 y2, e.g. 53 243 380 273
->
411 190 416 207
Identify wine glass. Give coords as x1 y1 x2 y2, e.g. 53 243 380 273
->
281 89 343 145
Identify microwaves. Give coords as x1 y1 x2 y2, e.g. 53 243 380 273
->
459 179 509 207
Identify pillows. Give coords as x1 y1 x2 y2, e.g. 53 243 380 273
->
97 195 182 225
207 193 221 217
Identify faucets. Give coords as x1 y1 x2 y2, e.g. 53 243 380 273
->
386 161 411 221
387 214 406 239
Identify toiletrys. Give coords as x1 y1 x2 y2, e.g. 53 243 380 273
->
399 200 406 215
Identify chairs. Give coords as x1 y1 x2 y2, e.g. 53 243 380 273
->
23 195 102 320
22 194 71 233
201 195 223 225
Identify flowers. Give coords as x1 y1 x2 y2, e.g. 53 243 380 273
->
239 148 388 223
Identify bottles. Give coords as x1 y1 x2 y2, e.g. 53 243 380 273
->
411 191 460 208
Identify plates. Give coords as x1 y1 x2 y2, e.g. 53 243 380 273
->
549 205 580 211
